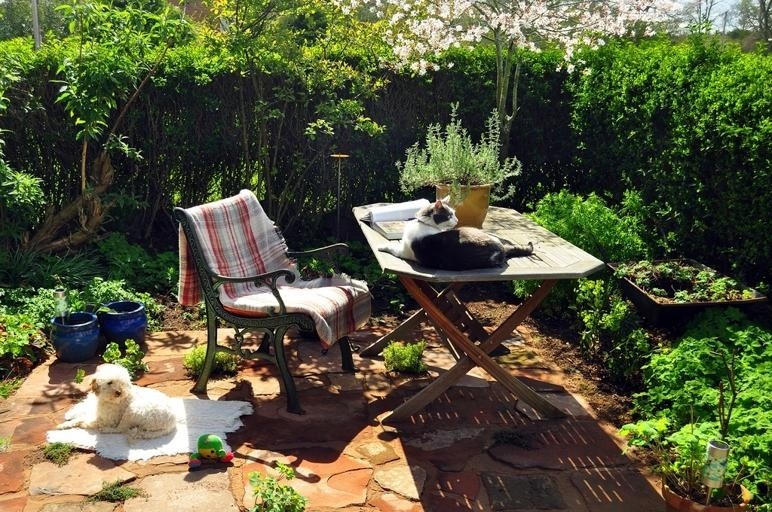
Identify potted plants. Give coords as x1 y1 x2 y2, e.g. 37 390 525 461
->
395 101 523 231
608 257 766 332
661 459 752 512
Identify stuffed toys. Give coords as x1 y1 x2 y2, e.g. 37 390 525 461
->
186 435 235 469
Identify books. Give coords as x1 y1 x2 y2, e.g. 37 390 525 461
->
359 197 431 241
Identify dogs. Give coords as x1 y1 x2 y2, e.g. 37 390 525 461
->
55 362 177 450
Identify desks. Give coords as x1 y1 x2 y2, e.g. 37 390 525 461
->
351 200 606 423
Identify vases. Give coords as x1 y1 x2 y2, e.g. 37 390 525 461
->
50 310 99 362
99 300 148 352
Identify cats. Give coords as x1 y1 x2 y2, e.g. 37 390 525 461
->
377 194 534 272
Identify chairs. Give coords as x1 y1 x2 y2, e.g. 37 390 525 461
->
172 188 372 413
